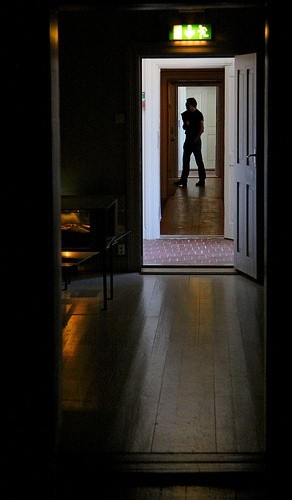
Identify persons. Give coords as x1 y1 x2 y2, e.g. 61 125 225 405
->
173 98 206 187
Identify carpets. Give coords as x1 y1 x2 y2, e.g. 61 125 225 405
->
143 238 234 265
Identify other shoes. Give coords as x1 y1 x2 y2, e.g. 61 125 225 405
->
173 177 188 187
195 179 206 186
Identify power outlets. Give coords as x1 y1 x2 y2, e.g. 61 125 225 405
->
118 244 126 256
